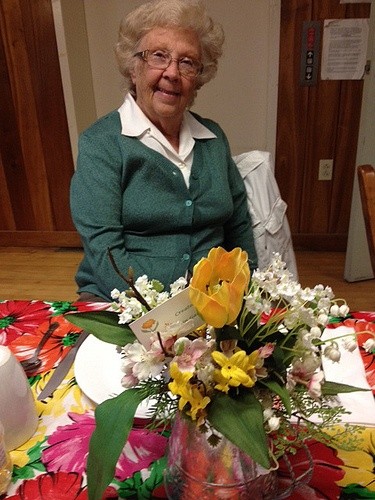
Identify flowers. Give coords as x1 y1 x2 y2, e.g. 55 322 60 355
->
64 246 375 500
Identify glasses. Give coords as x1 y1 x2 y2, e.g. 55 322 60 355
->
132 48 203 79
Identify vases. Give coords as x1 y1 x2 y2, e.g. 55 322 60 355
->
166 406 277 500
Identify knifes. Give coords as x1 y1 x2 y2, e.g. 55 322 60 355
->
37 329 89 400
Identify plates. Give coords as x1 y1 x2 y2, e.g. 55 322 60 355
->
73 334 177 420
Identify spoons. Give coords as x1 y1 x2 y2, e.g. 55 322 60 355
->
19 322 59 372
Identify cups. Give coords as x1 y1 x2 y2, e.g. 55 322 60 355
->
0 344 39 452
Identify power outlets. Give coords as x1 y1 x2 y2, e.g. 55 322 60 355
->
319 159 333 180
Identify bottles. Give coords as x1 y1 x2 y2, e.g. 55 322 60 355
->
0 424 14 496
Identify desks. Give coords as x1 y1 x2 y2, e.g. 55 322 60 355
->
0 299 375 500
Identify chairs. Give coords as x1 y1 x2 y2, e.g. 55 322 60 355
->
357 164 375 278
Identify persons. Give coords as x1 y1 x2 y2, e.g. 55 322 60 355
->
68 0 259 302
